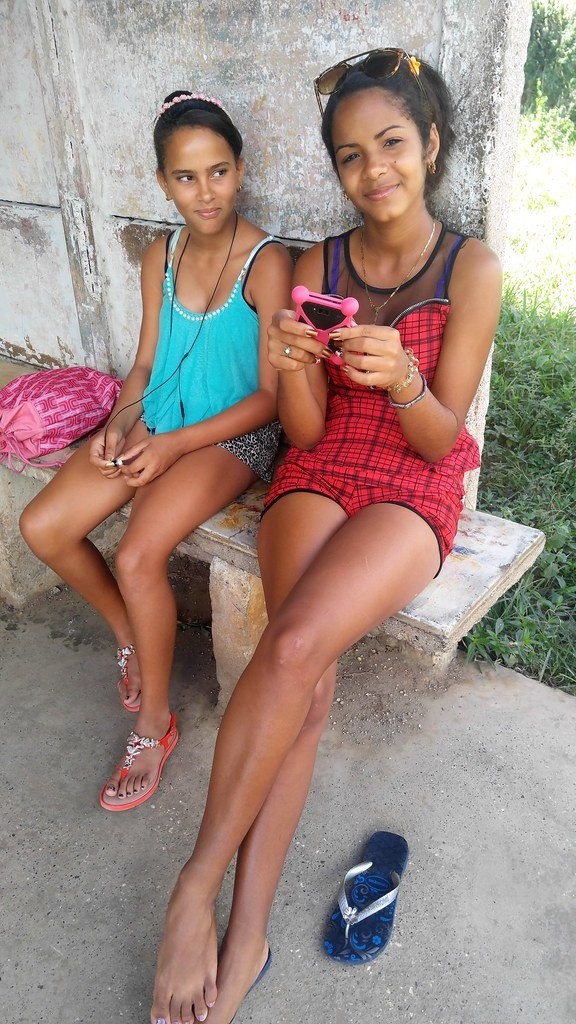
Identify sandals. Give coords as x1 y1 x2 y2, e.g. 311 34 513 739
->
100 709 178 812
117 645 140 712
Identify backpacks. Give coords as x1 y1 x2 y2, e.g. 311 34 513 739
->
0 366 125 474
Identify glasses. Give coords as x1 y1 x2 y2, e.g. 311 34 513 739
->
314 47 433 122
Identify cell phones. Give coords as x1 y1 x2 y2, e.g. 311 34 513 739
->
292 284 364 368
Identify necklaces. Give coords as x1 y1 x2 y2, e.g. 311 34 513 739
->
361 219 436 326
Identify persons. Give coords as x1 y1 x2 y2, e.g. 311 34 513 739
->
146 51 504 1024
18 91 297 812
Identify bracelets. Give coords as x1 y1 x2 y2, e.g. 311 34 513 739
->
388 372 428 409
381 348 420 394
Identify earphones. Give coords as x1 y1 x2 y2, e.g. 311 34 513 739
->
111 460 123 467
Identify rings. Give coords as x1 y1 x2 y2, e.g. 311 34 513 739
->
284 344 293 357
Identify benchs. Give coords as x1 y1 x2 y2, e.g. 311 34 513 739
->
2 354 543 726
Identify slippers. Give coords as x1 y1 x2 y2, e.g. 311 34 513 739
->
323 831 409 966
